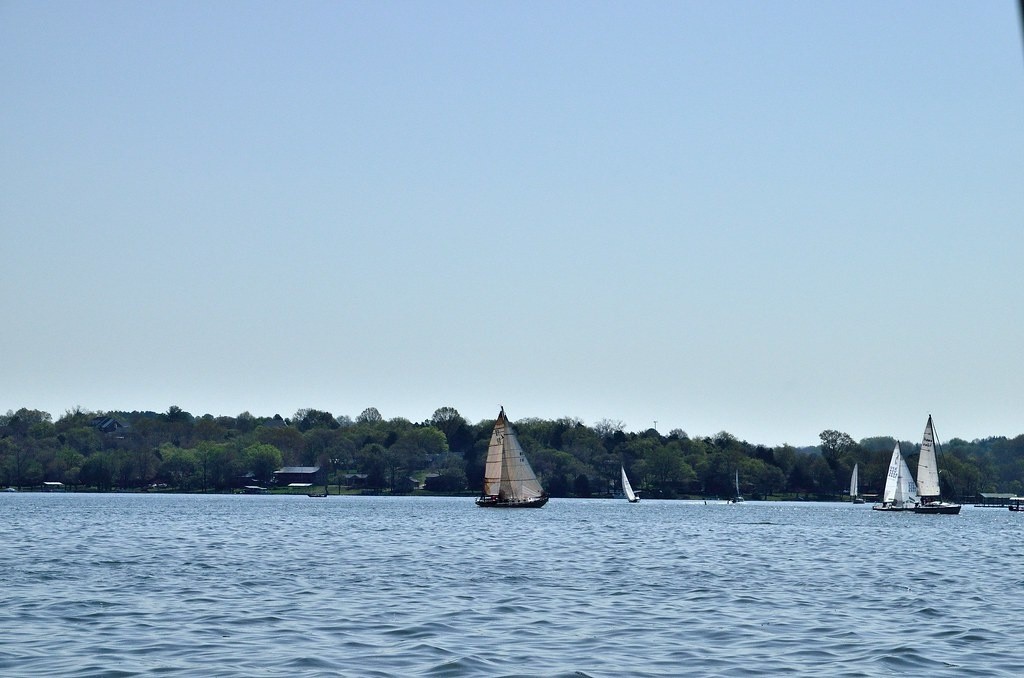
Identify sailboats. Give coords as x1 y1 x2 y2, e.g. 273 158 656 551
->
731 470 745 501
474 403 552 509
851 463 867 505
914 412 963 515
620 465 640 503
872 439 922 510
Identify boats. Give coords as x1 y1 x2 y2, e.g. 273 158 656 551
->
1008 496 1024 511
